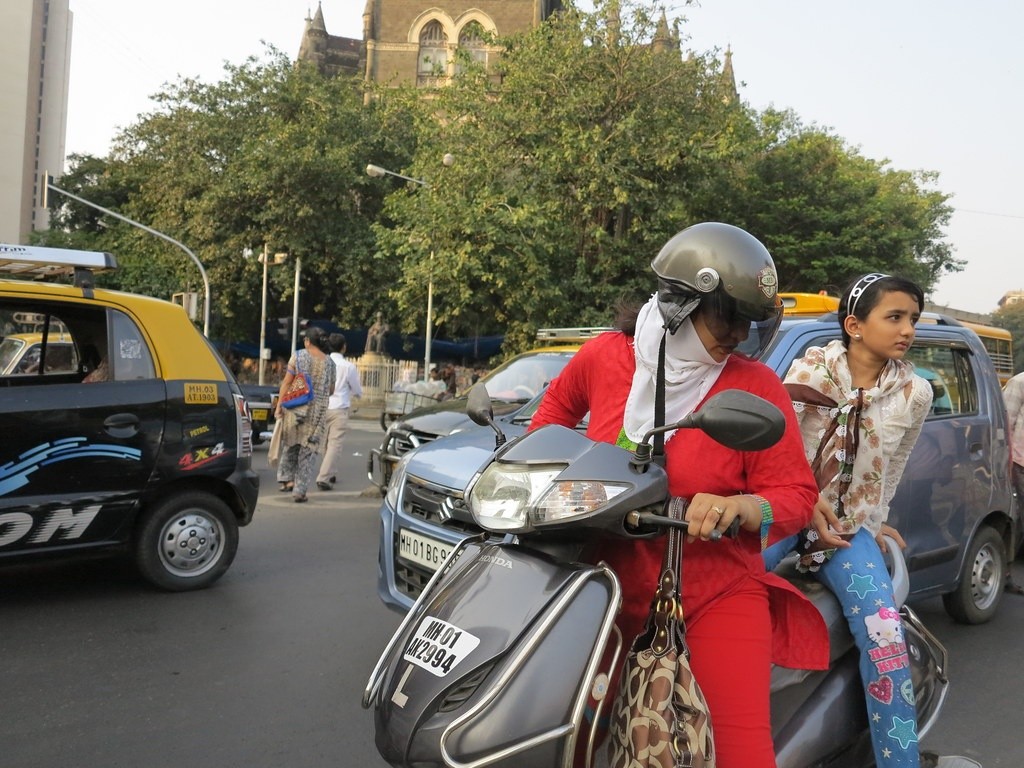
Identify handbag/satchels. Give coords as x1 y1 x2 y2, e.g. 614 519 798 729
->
281 349 314 409
606 496 716 768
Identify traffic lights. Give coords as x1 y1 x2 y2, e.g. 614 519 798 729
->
277 317 292 340
297 317 310 342
172 292 188 311
13 313 42 324
189 292 197 321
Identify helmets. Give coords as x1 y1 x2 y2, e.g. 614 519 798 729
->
650 221 784 362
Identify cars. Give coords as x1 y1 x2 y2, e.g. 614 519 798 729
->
0 322 78 376
367 327 624 499
0 242 261 591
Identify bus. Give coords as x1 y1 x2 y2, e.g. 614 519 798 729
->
778 290 1013 414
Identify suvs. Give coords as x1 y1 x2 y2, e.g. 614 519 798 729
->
377 312 1016 625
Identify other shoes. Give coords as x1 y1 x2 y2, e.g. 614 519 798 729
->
329 476 336 484
317 481 331 491
919 753 982 768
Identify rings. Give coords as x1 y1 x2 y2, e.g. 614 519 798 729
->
713 505 723 516
275 416 276 417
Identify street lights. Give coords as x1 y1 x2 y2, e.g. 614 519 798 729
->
273 253 300 353
258 243 268 385
443 153 480 358
367 164 437 382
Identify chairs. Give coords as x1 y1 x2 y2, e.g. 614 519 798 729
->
76 344 101 382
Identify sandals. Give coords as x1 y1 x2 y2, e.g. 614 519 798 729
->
294 495 308 503
278 481 293 492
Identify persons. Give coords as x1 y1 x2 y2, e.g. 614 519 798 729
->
274 326 336 502
224 352 285 385
315 333 361 491
17 346 73 374
1001 371 1024 534
410 361 483 399
526 221 830 768
759 275 935 768
363 309 389 354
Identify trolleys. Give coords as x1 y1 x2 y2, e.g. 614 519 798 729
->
380 390 441 432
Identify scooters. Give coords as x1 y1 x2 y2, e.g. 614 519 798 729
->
362 382 949 768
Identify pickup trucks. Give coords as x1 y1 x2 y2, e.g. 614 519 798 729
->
239 384 280 445
